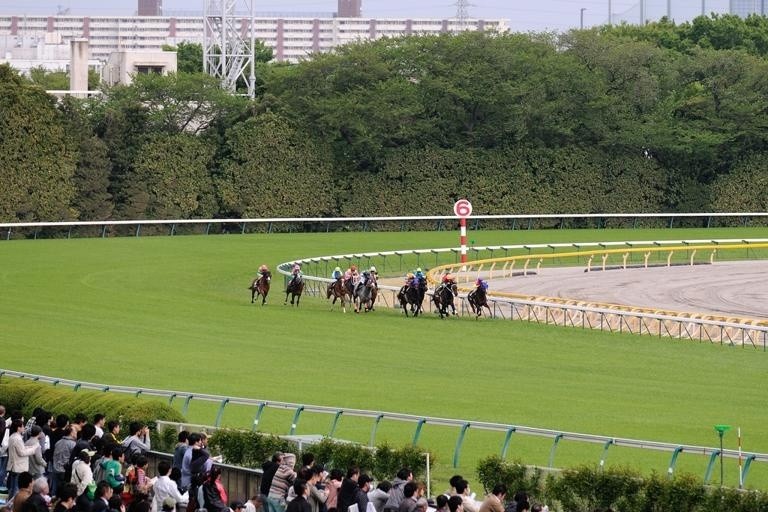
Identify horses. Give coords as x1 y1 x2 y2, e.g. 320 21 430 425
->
328 273 378 313
433 283 458 320
468 281 493 321
248 271 272 307
399 276 428 317
284 273 305 307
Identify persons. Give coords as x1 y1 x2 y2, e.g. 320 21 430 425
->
287 264 304 287
260 451 543 511
401 268 483 298
0 404 268 512
254 265 268 288
329 265 377 291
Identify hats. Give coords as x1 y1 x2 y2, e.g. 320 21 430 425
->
357 473 373 483
80 448 95 456
112 447 124 457
162 497 176 508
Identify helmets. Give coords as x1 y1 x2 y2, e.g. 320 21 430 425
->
407 273 412 278
262 265 266 269
295 265 300 269
334 266 377 277
478 277 483 281
417 268 421 272
